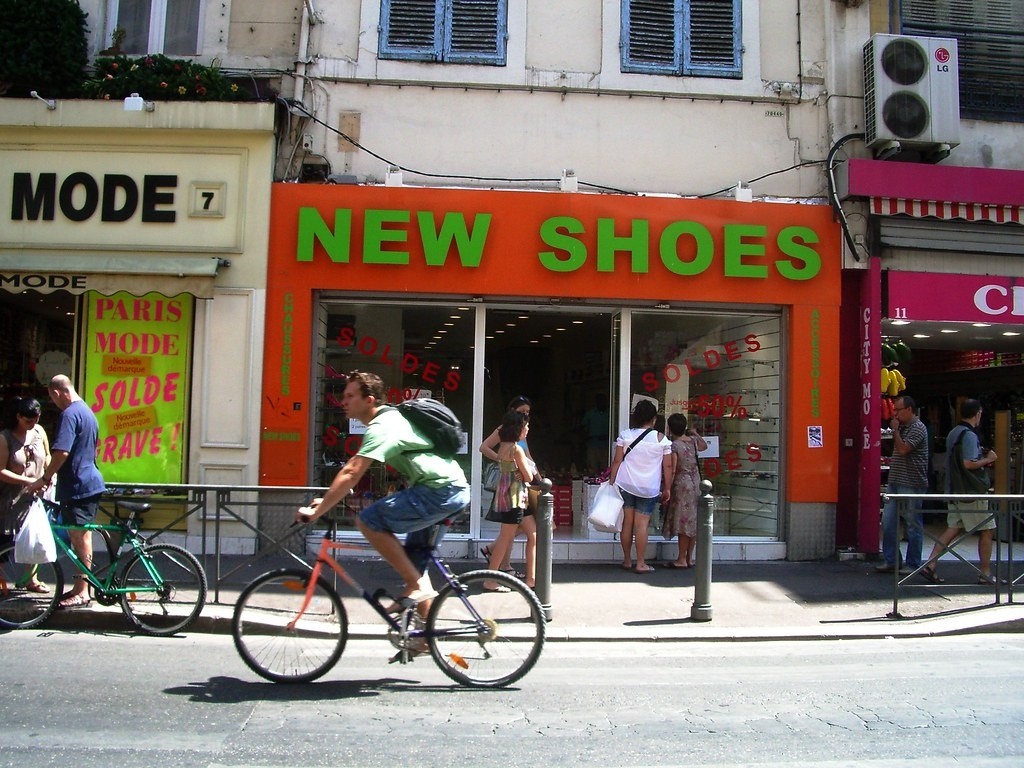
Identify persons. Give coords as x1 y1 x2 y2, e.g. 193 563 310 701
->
922 399 1008 585
875 396 928 574
295 372 472 664
480 395 543 592
662 413 708 568
610 400 672 572
582 392 610 477
0 396 52 593
28 374 107 608
707 441 711 444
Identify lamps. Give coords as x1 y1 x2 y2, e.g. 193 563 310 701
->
559 169 579 193
123 90 157 114
327 172 358 185
735 178 755 203
383 165 404 189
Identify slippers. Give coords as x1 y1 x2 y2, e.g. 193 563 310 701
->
531 585 536 590
662 561 688 569
620 562 633 570
383 586 439 615
634 565 656 573
686 562 696 568
482 583 512 594
387 643 431 664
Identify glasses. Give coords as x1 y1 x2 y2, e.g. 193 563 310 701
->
892 407 907 412
24 444 35 462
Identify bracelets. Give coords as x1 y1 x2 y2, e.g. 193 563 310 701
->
41 476 50 485
892 428 899 434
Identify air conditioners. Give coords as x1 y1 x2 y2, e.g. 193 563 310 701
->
858 31 962 151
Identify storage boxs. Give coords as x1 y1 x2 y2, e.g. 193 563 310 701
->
549 479 616 539
947 350 1024 371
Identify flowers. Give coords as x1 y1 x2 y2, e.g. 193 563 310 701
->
79 53 243 101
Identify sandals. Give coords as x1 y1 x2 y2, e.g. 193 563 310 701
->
499 568 527 580
919 567 945 584
480 545 492 564
977 574 1009 585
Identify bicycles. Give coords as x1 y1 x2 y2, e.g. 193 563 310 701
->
232 503 546 688
0 483 208 637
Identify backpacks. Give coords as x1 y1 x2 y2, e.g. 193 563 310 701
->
368 398 463 459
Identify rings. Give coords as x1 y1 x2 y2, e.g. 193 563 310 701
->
30 484 33 487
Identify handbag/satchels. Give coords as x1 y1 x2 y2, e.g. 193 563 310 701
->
587 482 625 534
13 498 57 564
950 429 991 504
484 462 498 491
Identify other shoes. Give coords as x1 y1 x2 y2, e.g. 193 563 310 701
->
899 564 922 574
26 582 51 593
52 590 94 610
875 563 903 574
